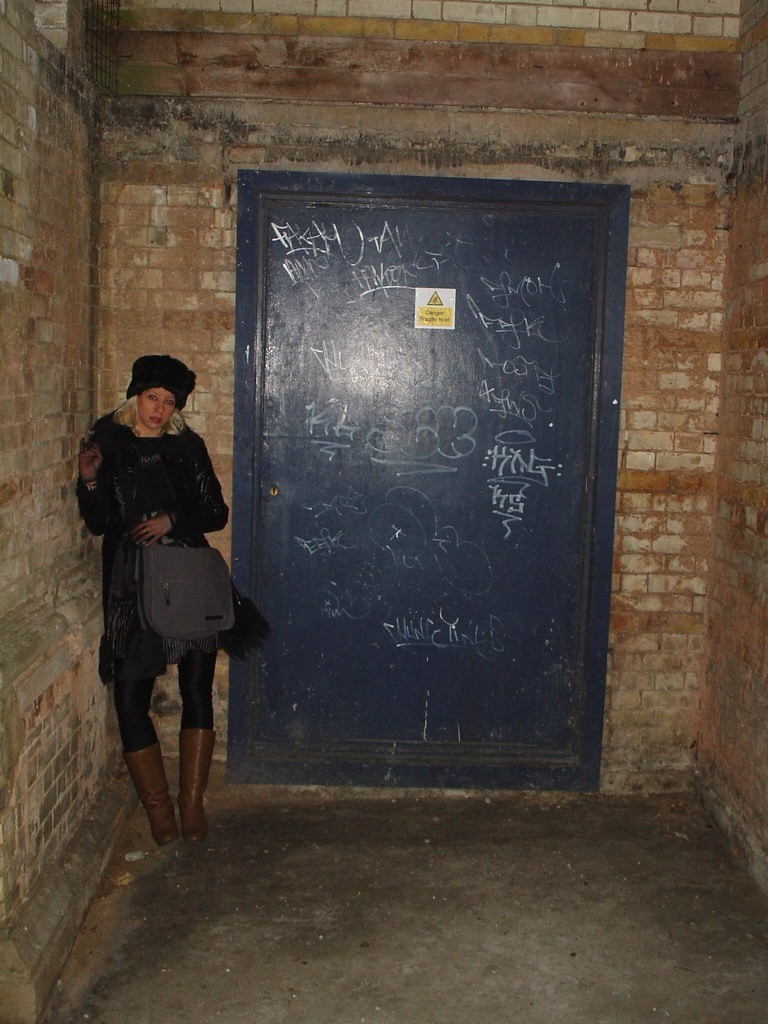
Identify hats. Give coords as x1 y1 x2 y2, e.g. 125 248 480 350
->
126 354 197 411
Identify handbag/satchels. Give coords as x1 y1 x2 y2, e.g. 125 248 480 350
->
135 510 235 640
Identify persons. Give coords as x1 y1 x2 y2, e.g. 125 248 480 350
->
76 356 271 844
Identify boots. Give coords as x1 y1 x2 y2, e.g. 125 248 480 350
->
177 728 216 846
122 740 180 846
